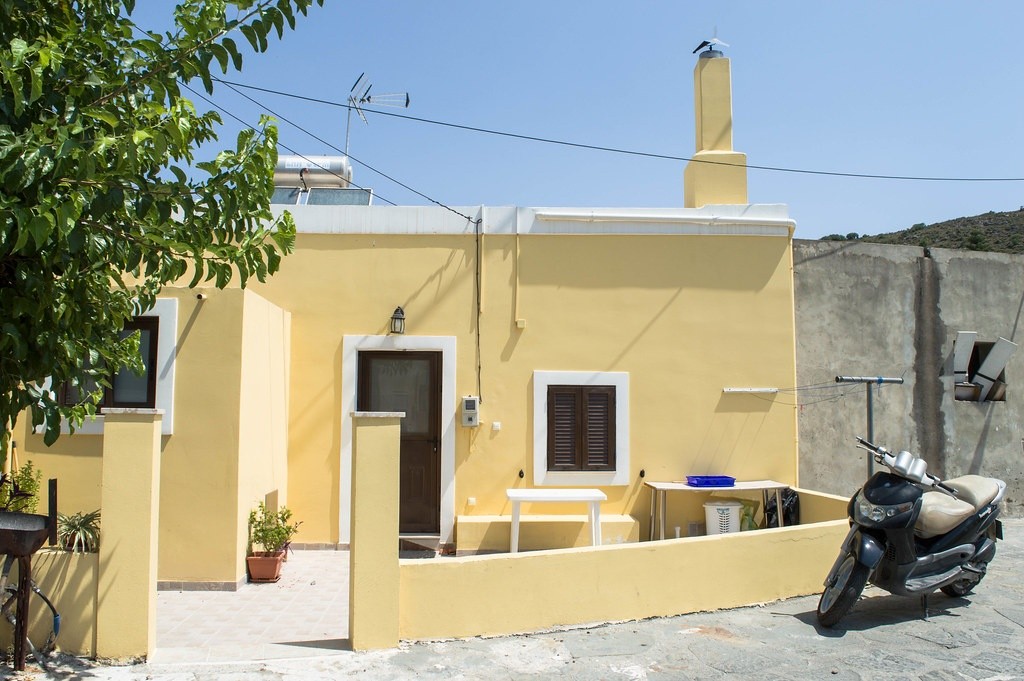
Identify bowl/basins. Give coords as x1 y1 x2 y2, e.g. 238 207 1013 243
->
955 382 984 402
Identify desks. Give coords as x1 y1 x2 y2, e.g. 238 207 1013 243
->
507 488 607 553
644 480 790 541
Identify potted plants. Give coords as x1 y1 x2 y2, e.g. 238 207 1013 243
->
274 520 302 562
57 508 101 553
246 499 297 583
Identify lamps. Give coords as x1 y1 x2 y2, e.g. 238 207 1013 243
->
390 306 405 334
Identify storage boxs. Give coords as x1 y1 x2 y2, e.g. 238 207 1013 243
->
686 475 736 487
702 500 745 537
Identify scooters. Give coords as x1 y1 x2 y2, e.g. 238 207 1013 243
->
814 435 1007 630
